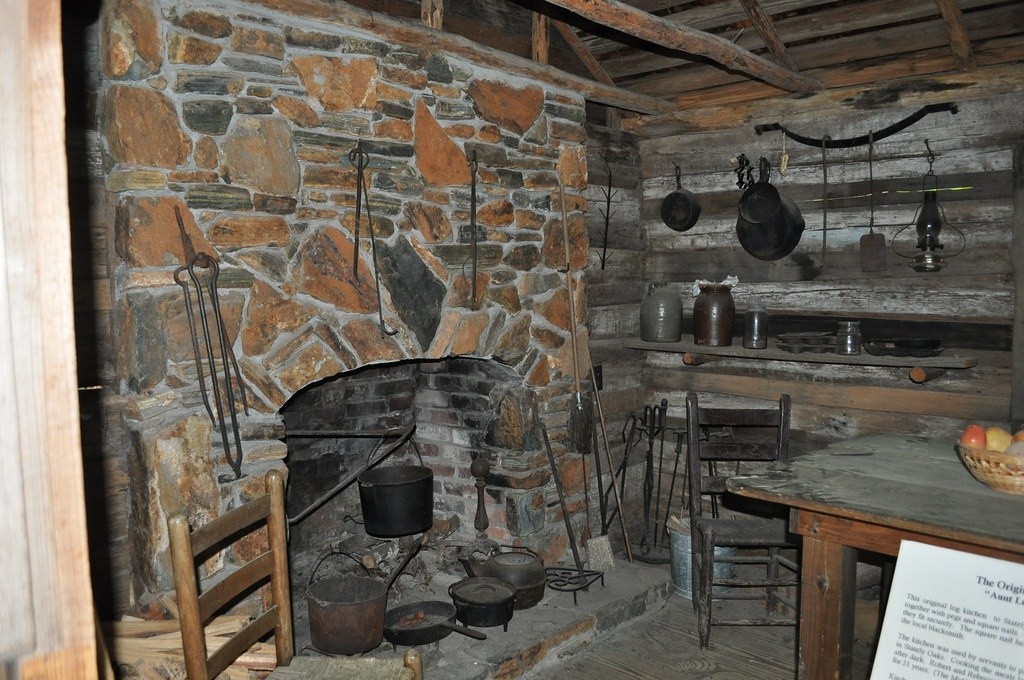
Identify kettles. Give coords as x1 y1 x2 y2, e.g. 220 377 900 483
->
458 546 546 611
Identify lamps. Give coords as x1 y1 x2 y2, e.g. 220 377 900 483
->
891 153 966 272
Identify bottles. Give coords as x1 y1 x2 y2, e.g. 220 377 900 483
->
639 280 682 342
836 321 862 355
692 283 736 346
743 303 770 349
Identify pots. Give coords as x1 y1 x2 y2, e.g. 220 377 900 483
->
661 165 701 231
383 600 487 646
449 577 516 632
735 193 806 260
738 158 781 224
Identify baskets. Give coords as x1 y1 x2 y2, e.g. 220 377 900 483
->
958 440 1024 495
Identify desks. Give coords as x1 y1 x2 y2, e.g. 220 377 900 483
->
725 432 1024 680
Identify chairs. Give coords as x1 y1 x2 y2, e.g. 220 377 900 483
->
168 469 424 680
685 394 805 649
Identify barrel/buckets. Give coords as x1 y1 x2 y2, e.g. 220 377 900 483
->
358 429 434 537
665 517 737 601
306 552 389 654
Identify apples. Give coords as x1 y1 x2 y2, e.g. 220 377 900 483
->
959 423 1024 453
